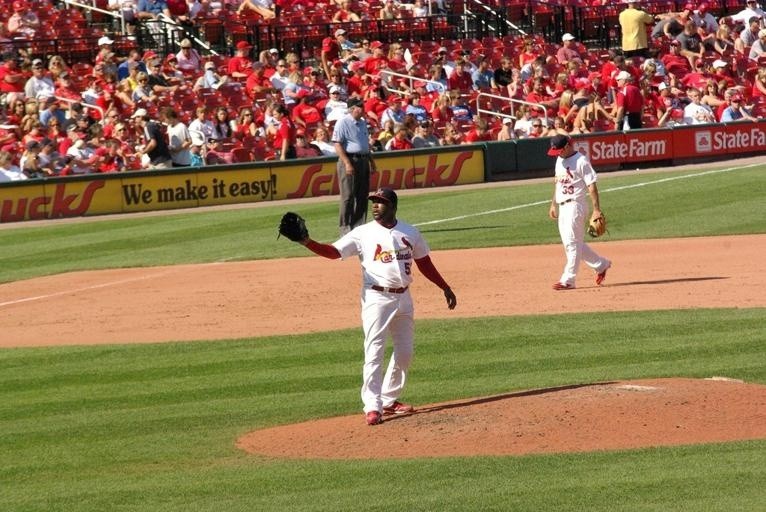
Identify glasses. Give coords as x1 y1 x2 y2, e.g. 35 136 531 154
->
139 78 147 83
109 115 119 118
117 127 126 131
134 59 141 62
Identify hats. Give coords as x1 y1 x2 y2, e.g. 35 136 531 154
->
32 58 42 66
503 119 512 124
561 32 576 42
59 71 70 79
72 104 82 110
1 138 55 153
615 71 631 80
130 108 148 119
367 188 397 207
733 95 741 102
46 95 60 105
685 4 708 12
546 134 570 157
658 82 673 93
321 29 347 53
406 62 419 72
204 61 215 70
370 41 384 49
388 95 405 102
348 97 364 108
166 54 177 63
420 120 430 127
98 36 114 46
749 16 762 23
695 58 728 69
296 89 311 99
236 41 253 50
330 87 340 94
478 53 488 63
270 49 278 54
143 51 158 59
671 39 681 46
431 47 447 65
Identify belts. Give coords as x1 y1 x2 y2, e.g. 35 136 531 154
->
347 152 370 159
560 199 571 206
372 284 409 293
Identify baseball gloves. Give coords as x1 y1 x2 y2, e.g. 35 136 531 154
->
587 213 606 236
279 211 308 244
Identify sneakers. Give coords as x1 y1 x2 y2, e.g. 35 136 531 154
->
596 261 612 285
366 410 380 425
382 402 414 414
553 282 570 290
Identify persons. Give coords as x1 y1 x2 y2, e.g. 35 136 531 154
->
332 97 378 234
280 186 455 424
547 135 615 289
1 1 766 182
